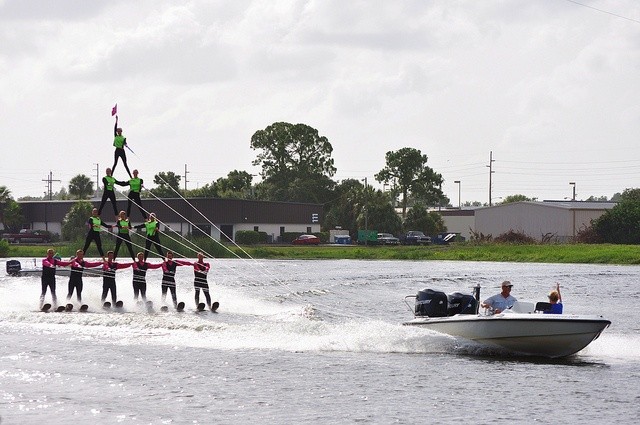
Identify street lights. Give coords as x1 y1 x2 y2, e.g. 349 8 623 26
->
489 196 502 206
564 196 573 201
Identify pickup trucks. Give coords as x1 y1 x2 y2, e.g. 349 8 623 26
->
399 231 432 245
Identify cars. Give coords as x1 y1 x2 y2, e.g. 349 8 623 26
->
2 228 46 243
377 233 400 245
292 234 321 245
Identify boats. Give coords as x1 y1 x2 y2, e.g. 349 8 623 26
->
5 259 104 278
402 283 612 360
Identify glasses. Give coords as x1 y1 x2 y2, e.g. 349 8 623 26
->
506 286 511 289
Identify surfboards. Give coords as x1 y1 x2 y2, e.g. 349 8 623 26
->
65 303 74 313
136 300 142 307
30 304 50 313
175 302 185 312
101 301 110 310
144 300 152 308
197 302 206 312
78 304 88 312
209 302 220 311
53 305 64 312
159 305 169 312
114 300 123 308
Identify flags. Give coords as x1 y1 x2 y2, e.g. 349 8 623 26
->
112 104 117 116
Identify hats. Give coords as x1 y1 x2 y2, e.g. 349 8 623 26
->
547 291 559 299
502 280 513 287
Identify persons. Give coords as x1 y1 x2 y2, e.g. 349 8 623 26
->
98 168 127 219
112 210 136 263
481 281 517 314
133 252 162 304
194 252 211 309
162 251 194 309
66 249 104 307
111 115 134 179
121 170 149 222
132 213 166 262
39 249 72 310
543 283 563 314
83 208 116 262
101 250 133 307
